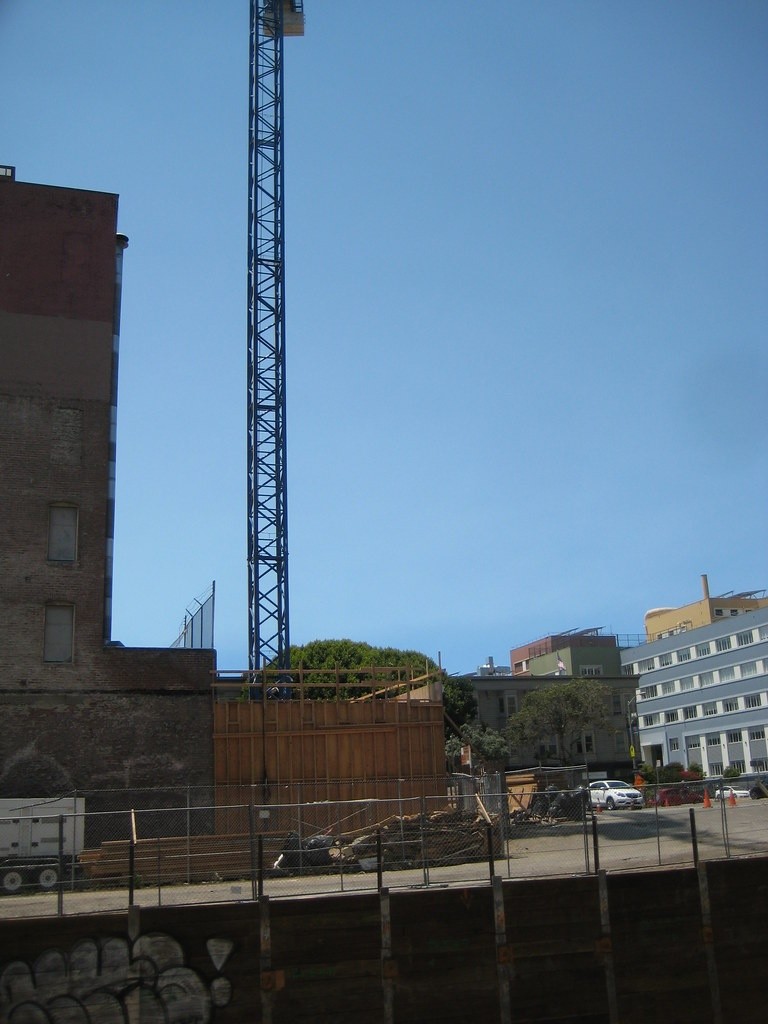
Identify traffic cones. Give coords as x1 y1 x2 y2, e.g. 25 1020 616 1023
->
595 797 603 813
664 798 671 807
727 788 737 807
701 788 714 809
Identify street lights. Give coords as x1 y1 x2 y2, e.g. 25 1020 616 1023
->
627 691 646 767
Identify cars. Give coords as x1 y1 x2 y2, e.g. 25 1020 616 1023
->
715 785 750 800
750 780 768 800
589 780 645 810
656 786 703 807
